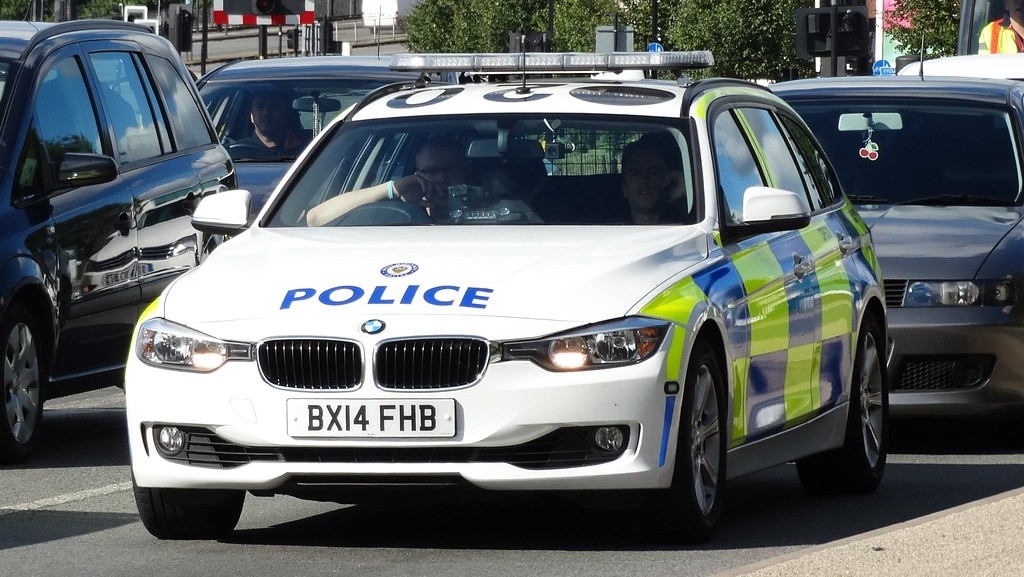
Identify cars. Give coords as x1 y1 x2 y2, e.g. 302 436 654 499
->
755 72 1024 429
192 53 462 240
119 51 892 546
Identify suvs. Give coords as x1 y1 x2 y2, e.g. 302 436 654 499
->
0 16 240 463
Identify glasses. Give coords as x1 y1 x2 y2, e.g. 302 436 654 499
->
417 161 468 181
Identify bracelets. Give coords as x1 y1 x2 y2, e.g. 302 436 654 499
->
388 180 394 199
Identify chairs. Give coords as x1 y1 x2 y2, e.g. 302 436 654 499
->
463 139 549 227
605 129 699 225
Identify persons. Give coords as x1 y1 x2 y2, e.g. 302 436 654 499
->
222 89 309 160
620 142 687 225
978 0 1024 55
306 135 496 227
